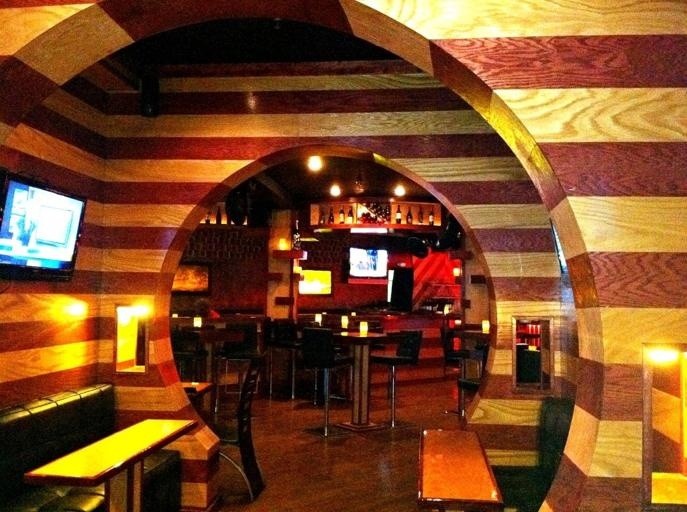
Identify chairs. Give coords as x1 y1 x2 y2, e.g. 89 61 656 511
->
265 319 461 439
171 319 266 495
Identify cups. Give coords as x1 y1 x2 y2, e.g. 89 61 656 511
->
358 321 368 335
481 320 489 334
340 315 348 329
192 317 202 327
444 306 449 315
314 313 322 327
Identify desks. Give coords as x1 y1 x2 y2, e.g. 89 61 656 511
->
24 418 198 512
170 317 460 501
416 428 504 512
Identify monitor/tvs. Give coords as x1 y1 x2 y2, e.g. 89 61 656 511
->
349 248 388 278
299 269 332 296
548 216 569 275
0 168 87 285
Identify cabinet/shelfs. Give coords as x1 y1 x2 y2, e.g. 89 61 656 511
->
306 197 446 232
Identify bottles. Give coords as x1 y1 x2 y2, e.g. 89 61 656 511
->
327 207 334 224
406 207 412 224
338 205 344 224
215 206 221 224
418 208 423 224
318 207 325 227
292 220 301 250
428 207 433 226
395 205 401 224
347 206 354 224
205 207 210 225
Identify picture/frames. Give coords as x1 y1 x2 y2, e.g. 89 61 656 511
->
171 262 212 296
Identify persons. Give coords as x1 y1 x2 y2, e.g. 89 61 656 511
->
193 297 224 352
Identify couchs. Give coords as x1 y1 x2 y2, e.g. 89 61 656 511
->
0 384 181 512
